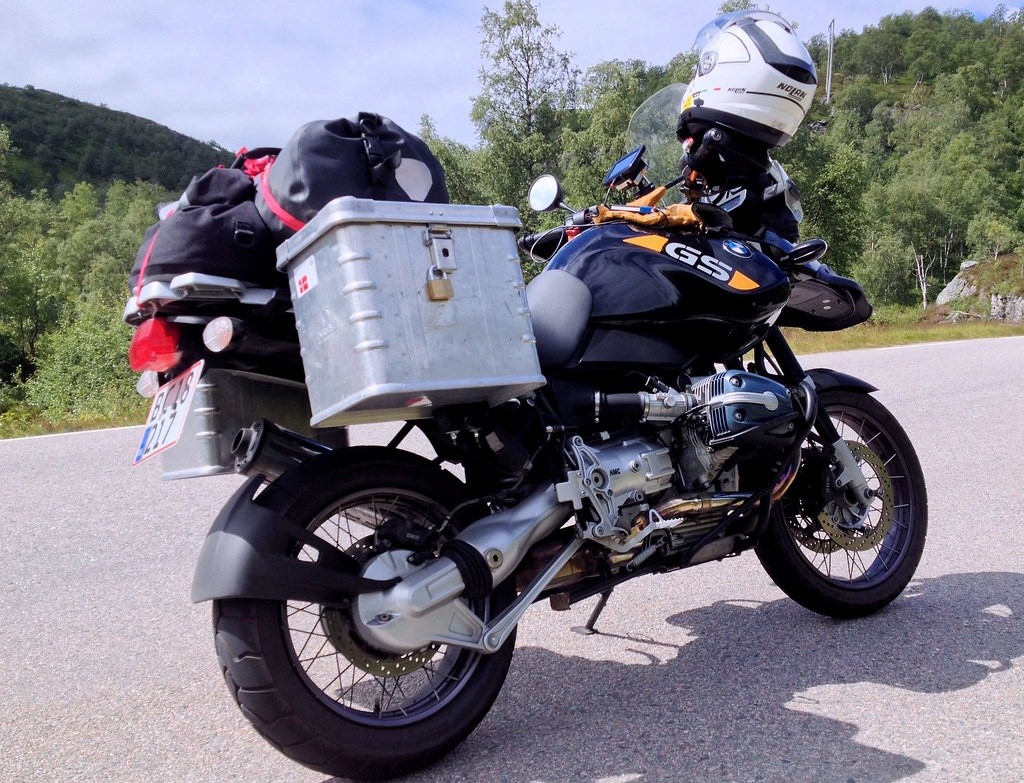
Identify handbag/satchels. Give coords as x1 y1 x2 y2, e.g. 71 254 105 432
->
130 112 450 292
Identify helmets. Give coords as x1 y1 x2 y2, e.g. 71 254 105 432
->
677 12 817 145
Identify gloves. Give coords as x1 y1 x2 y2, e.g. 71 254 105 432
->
666 202 732 233
591 185 693 227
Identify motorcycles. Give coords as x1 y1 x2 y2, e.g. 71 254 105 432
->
116 83 930 783
